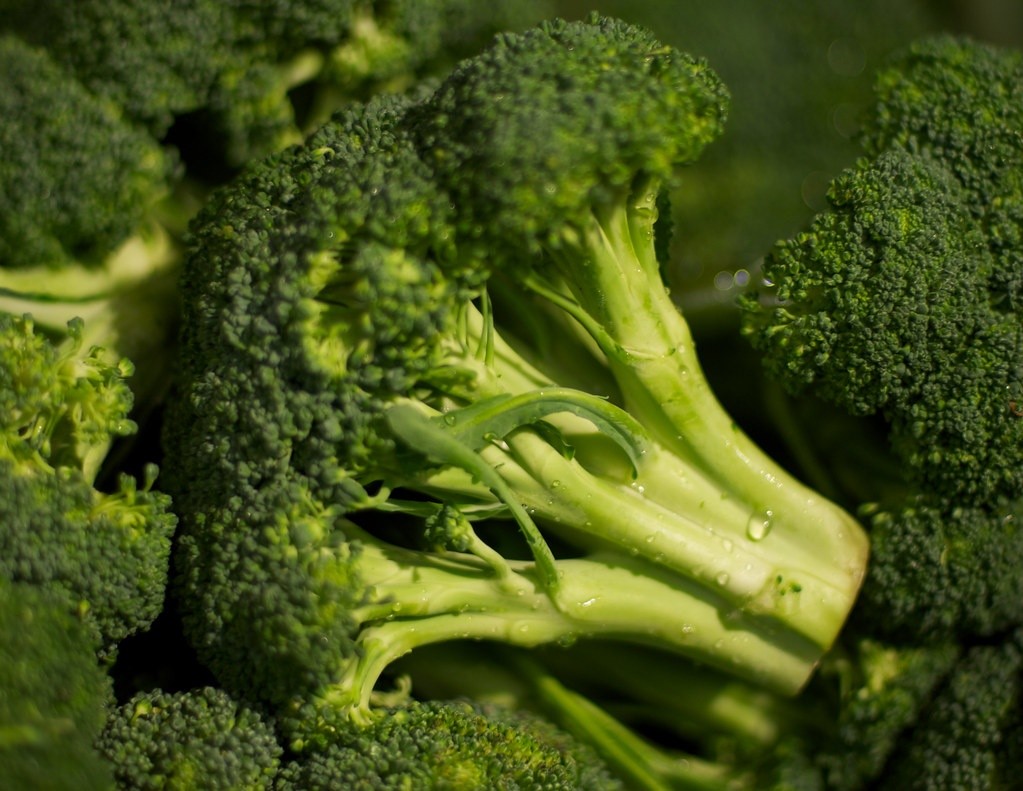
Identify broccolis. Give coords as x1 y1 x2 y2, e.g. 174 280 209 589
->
0 0 1023 791
